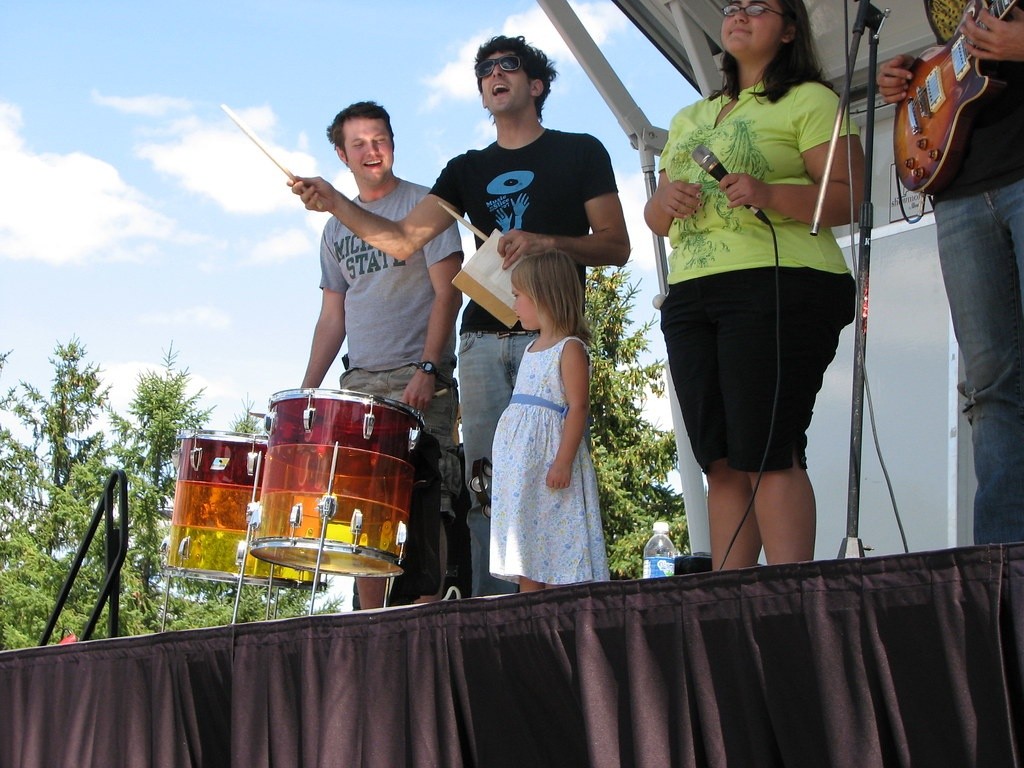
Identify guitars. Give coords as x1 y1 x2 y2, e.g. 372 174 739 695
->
892 0 1017 197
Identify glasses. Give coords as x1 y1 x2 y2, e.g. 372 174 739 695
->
476 54 521 78
720 4 783 16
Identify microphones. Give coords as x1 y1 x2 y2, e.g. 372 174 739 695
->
692 144 771 227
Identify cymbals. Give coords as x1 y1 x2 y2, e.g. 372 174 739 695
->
467 456 492 520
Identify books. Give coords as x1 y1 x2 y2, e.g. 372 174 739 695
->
452 229 521 329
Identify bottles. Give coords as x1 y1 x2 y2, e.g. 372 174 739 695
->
643 522 675 579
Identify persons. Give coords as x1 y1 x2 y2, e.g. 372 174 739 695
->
303 102 468 606
288 34 631 597
877 0 1024 545
643 0 866 570
489 251 610 592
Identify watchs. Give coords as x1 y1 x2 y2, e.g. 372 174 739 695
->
417 361 437 374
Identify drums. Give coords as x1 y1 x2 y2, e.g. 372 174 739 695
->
247 387 427 579
166 427 328 593
